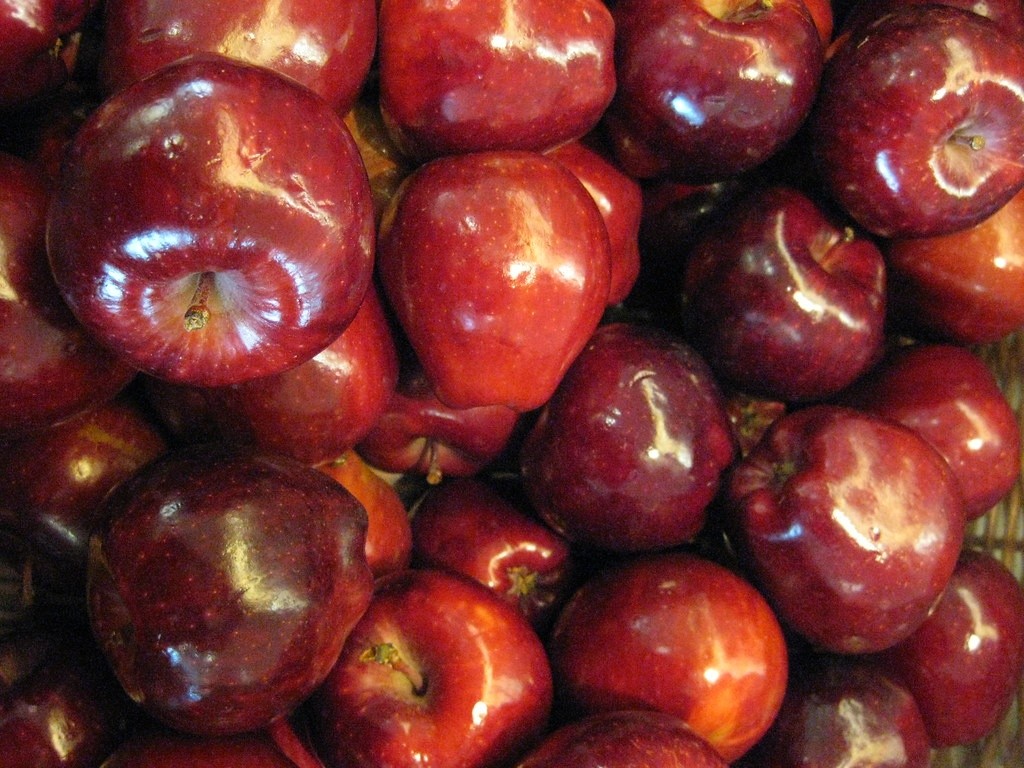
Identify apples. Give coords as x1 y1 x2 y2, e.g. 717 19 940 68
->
0 0 1024 768
